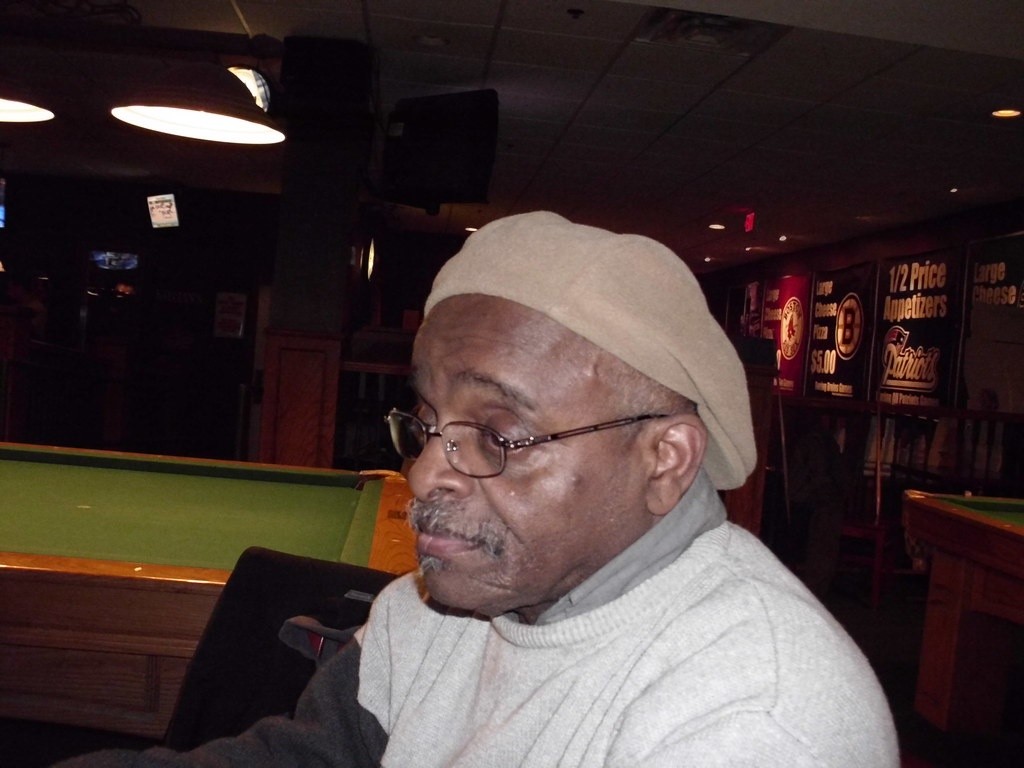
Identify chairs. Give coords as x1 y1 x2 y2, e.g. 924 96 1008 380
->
843 401 1024 611
164 546 399 754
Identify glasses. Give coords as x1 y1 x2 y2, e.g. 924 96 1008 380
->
383 407 668 477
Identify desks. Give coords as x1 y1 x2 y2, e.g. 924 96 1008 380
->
901 490 1023 736
0 442 421 741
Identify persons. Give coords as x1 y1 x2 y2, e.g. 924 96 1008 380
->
47 210 902 768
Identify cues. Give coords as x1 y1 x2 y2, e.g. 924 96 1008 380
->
773 367 791 526
874 389 882 530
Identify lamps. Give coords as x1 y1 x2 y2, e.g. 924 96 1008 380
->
110 63 289 144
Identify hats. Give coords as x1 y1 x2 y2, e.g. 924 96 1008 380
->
423 210 757 489
